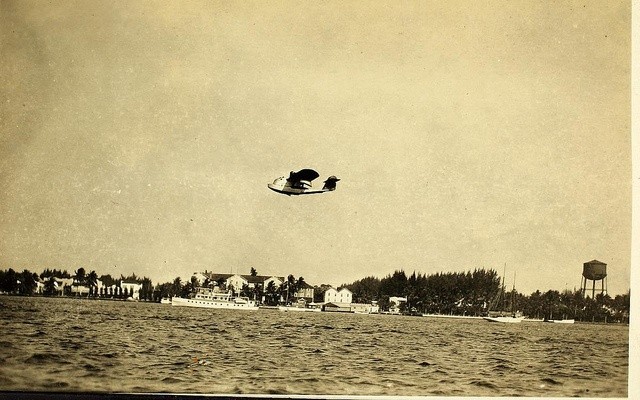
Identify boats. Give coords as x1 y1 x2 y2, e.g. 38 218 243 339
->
548 319 574 323
278 304 321 312
482 315 525 322
523 318 544 321
354 306 369 313
160 297 171 304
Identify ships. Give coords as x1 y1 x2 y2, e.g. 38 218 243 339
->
171 288 259 311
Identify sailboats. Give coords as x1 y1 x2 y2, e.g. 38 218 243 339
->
488 262 523 316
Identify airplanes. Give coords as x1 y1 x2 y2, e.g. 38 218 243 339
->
268 169 340 196
